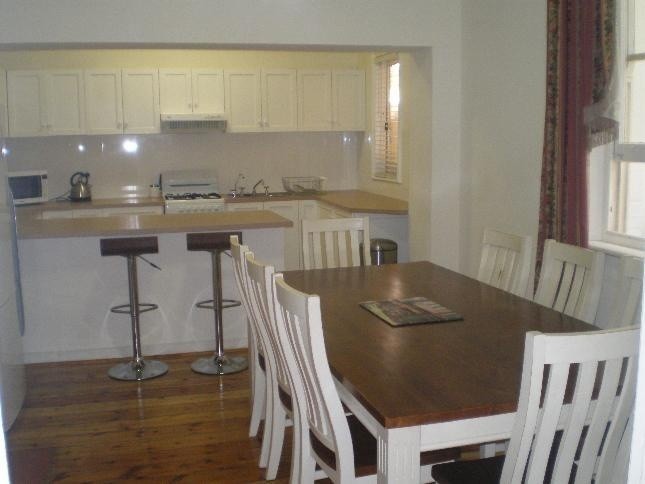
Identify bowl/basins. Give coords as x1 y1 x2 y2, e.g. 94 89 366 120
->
282 176 325 196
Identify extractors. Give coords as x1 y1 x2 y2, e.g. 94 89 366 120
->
160 116 229 136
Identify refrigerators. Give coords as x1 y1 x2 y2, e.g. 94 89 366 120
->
0 130 28 433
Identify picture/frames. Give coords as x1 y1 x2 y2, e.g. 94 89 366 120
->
359 297 464 327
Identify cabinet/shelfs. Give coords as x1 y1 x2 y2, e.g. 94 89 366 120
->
83 43 160 133
225 44 298 132
160 43 225 121
227 200 300 270
318 201 409 267
300 201 319 270
0 43 82 135
298 44 372 131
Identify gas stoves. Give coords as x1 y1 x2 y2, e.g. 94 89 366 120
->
159 170 227 213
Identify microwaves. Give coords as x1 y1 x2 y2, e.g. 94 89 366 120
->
7 169 48 205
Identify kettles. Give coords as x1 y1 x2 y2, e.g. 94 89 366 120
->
69 170 91 202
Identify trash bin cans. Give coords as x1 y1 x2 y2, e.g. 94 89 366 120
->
359 238 397 267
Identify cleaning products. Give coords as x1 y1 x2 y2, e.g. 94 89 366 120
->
235 172 248 196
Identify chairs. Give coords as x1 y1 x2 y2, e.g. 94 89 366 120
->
477 228 533 297
271 273 454 484
242 251 300 484
603 255 644 330
532 239 605 323
229 236 274 468
302 216 371 269
431 324 639 484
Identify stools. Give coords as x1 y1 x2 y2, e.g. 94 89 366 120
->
100 236 169 381
186 232 249 375
